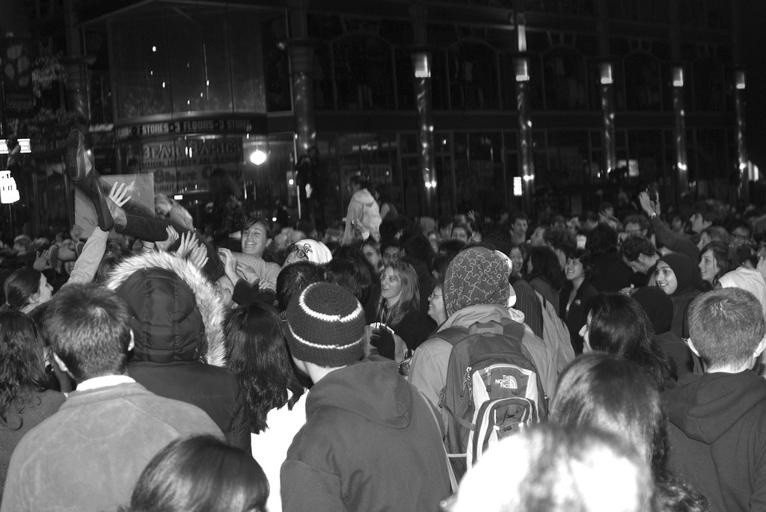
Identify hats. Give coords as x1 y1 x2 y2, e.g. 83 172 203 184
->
294 237 333 265
283 287 367 366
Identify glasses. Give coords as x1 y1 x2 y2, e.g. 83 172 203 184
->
291 243 306 259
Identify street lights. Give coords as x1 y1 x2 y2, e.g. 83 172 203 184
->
0 134 34 242
250 130 301 222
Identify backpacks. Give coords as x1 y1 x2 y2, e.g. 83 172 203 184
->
432 319 550 489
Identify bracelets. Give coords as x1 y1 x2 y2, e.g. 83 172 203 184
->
651 213 657 217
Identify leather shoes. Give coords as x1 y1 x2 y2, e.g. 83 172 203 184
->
90 177 114 232
66 128 92 182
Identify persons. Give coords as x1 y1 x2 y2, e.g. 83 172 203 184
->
2 173 765 510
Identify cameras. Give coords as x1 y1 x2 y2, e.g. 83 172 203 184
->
647 184 657 201
